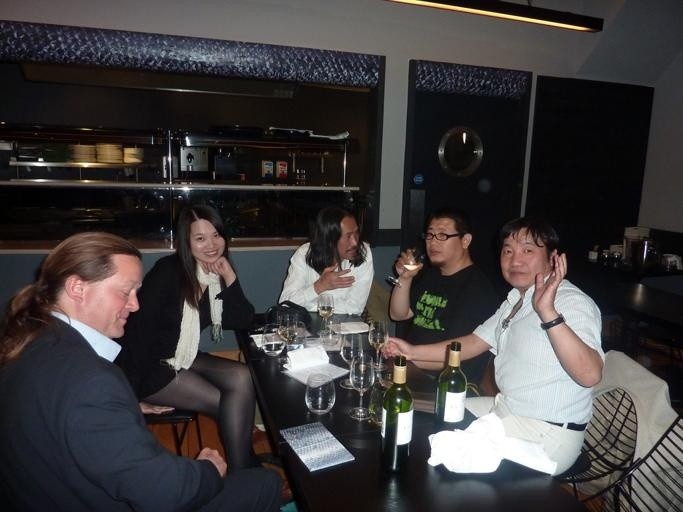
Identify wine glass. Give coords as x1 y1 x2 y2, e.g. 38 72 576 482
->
317 294 335 336
386 246 427 288
340 334 364 390
349 360 372 421
367 321 388 372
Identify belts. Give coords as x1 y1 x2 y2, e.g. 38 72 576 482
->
547 420 587 431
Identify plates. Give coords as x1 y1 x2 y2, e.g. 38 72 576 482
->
97 143 122 163
71 144 96 163
123 147 144 163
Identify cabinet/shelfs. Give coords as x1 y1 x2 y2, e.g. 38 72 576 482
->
0 58 371 256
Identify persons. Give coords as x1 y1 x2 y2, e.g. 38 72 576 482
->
1 232 284 512
390 213 495 387
382 217 605 476
114 206 264 474
279 205 374 315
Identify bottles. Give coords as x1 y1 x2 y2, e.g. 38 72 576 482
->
379 355 414 473
434 341 468 430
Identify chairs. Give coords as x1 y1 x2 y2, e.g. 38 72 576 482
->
139 400 202 456
581 411 683 512
550 348 667 501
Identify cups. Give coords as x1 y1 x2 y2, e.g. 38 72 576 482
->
321 316 339 347
613 251 621 268
290 321 309 350
263 323 288 356
368 381 392 426
267 307 292 333
602 249 611 268
304 375 336 419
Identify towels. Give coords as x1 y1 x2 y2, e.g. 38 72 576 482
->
427 415 507 473
332 322 372 335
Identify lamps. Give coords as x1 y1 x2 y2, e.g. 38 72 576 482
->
384 0 603 33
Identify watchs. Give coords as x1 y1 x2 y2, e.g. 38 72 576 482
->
541 314 565 329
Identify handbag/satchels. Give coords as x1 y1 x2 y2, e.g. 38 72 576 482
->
265 302 310 328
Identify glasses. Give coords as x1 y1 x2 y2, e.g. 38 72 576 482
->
421 232 460 241
